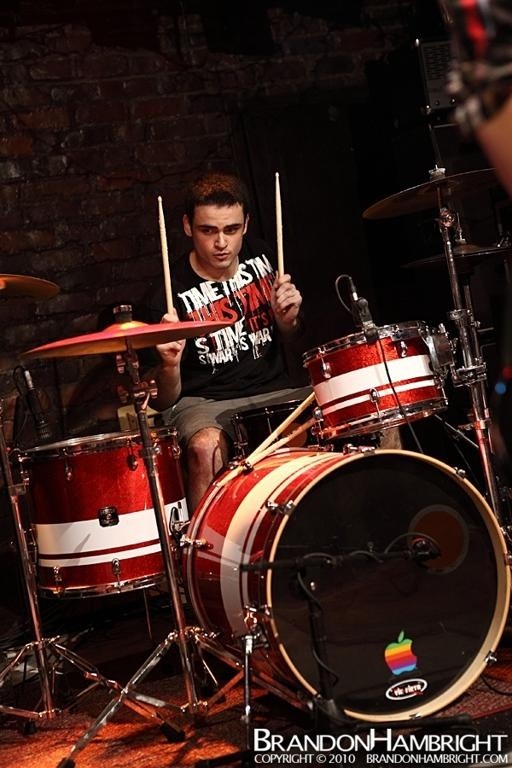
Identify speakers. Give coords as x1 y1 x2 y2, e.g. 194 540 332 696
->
201 0 285 56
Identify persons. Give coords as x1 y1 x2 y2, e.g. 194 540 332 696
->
142 173 309 526
436 0 512 204
59 301 147 437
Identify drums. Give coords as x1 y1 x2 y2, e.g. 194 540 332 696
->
13 426 190 600
183 446 512 732
232 389 381 452
300 322 448 440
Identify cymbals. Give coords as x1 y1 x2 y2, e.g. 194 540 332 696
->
398 244 511 265
360 166 495 223
1 274 57 304
18 305 233 358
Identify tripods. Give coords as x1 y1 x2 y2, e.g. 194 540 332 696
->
57 382 313 768
0 441 187 741
